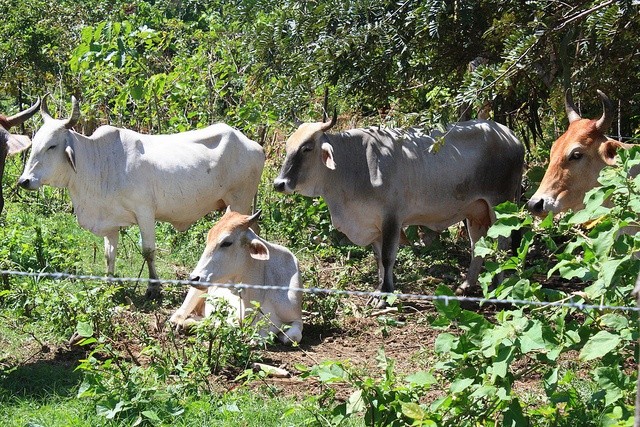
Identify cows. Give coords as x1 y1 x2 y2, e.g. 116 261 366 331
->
525 86 640 220
272 105 525 308
0 94 41 213
18 91 266 301
167 203 304 349
